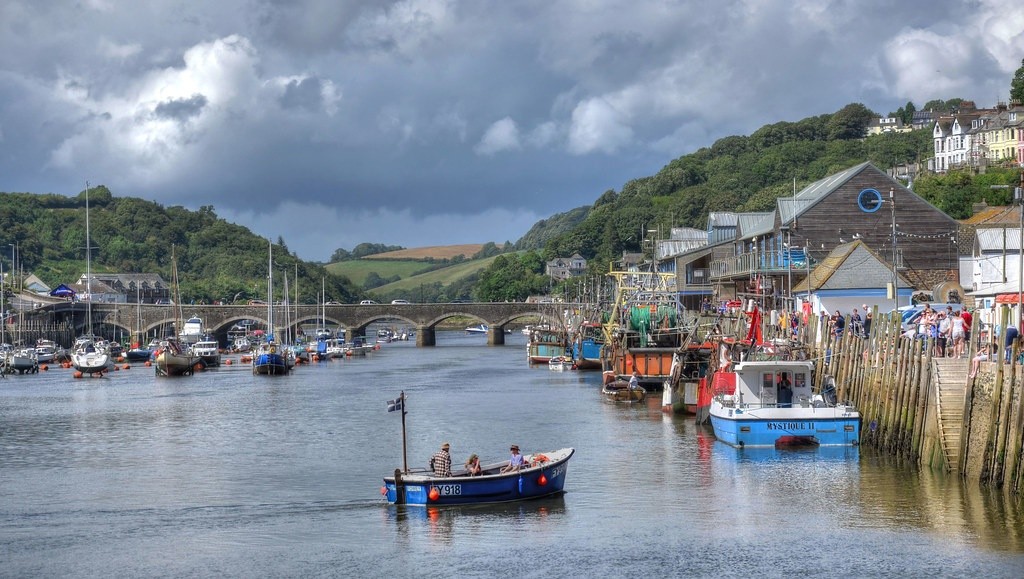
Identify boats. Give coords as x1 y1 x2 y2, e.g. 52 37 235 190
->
521 304 863 451
383 447 576 508
464 323 512 335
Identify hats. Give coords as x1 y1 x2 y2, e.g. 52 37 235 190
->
511 445 519 450
469 453 478 461
442 443 450 449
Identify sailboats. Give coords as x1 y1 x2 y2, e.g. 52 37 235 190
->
0 180 414 381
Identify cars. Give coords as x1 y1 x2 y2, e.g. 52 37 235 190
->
321 302 343 305
249 300 268 305
391 299 410 304
359 300 378 304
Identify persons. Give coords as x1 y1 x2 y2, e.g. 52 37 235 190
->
191 296 231 306
465 453 483 476
777 372 791 390
429 443 452 477
702 297 1018 365
500 445 523 474
627 371 638 390
